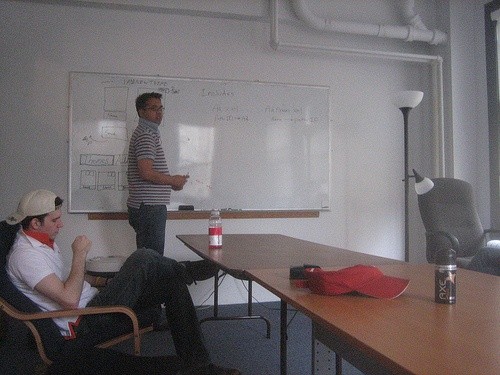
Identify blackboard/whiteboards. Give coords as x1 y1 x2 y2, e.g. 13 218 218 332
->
67 70 334 213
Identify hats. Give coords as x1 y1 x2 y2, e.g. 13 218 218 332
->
308 264 411 300
6 188 64 225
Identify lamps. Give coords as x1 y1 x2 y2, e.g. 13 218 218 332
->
391 90 434 263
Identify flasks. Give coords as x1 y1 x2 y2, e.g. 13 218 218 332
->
434 248 458 304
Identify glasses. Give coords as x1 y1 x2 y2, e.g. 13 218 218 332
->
144 106 166 113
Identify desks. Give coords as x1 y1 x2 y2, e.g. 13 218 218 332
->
245 264 500 375
176 234 410 338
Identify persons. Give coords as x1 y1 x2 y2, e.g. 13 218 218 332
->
5 189 241 375
126 92 190 331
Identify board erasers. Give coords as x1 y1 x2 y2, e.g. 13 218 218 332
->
179 204 195 210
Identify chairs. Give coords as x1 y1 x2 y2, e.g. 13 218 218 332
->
0 298 153 375
418 178 500 276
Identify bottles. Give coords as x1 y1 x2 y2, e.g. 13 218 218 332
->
207 210 223 249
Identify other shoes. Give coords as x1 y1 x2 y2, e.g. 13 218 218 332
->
206 363 241 375
182 259 220 282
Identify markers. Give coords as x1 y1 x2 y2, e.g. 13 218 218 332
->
185 171 190 178
